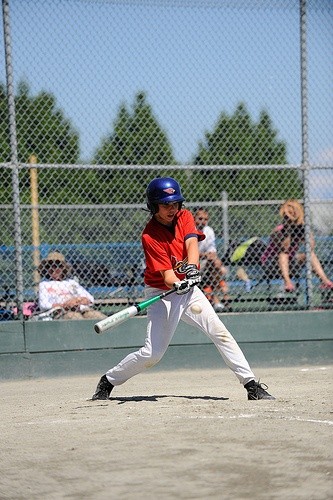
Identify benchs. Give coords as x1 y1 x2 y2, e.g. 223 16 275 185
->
0 235 333 306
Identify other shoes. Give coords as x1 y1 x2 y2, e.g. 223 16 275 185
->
319 283 333 291
243 279 252 293
285 285 294 292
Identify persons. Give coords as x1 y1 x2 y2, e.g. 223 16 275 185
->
93 177 277 400
37 252 107 320
262 199 333 292
190 205 241 312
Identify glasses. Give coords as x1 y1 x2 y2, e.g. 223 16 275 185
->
197 217 208 222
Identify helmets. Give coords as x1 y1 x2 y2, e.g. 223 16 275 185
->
146 178 185 214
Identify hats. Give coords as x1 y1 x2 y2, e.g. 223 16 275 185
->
37 252 69 276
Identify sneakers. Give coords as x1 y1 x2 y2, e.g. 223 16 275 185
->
92 375 114 399
248 377 275 400
214 303 224 312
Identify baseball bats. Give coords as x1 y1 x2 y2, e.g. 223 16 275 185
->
94 286 176 334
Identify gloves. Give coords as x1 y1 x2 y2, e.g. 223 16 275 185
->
173 281 191 296
185 264 202 287
